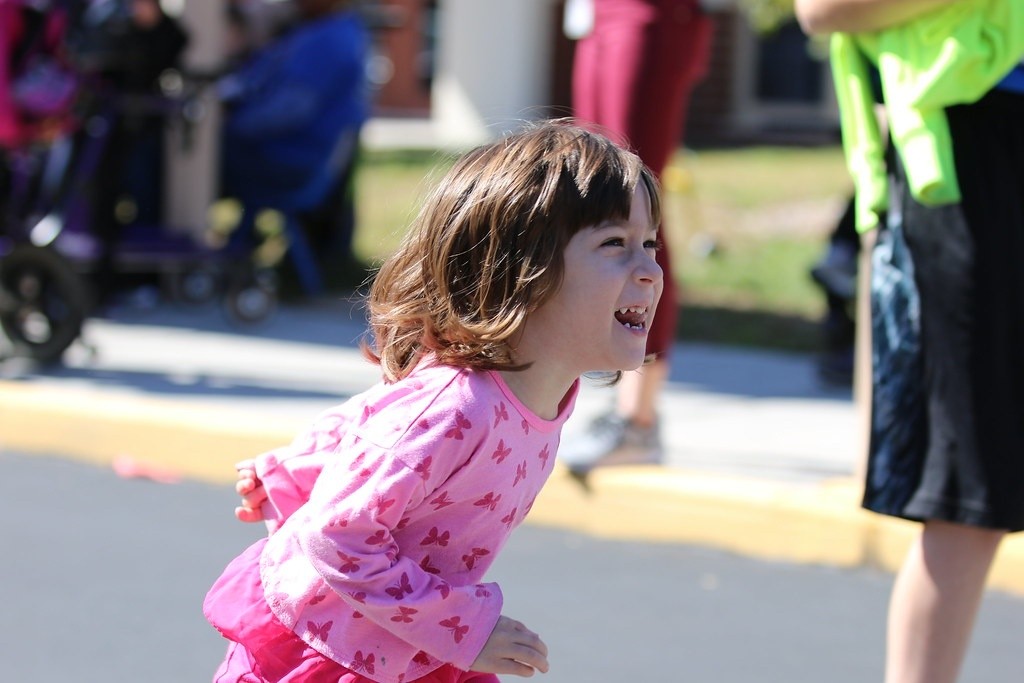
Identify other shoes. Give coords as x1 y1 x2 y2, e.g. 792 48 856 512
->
553 403 661 470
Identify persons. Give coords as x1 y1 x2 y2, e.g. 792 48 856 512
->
794 0 1024 683
560 0 715 471
0 0 364 326
202 117 664 683
